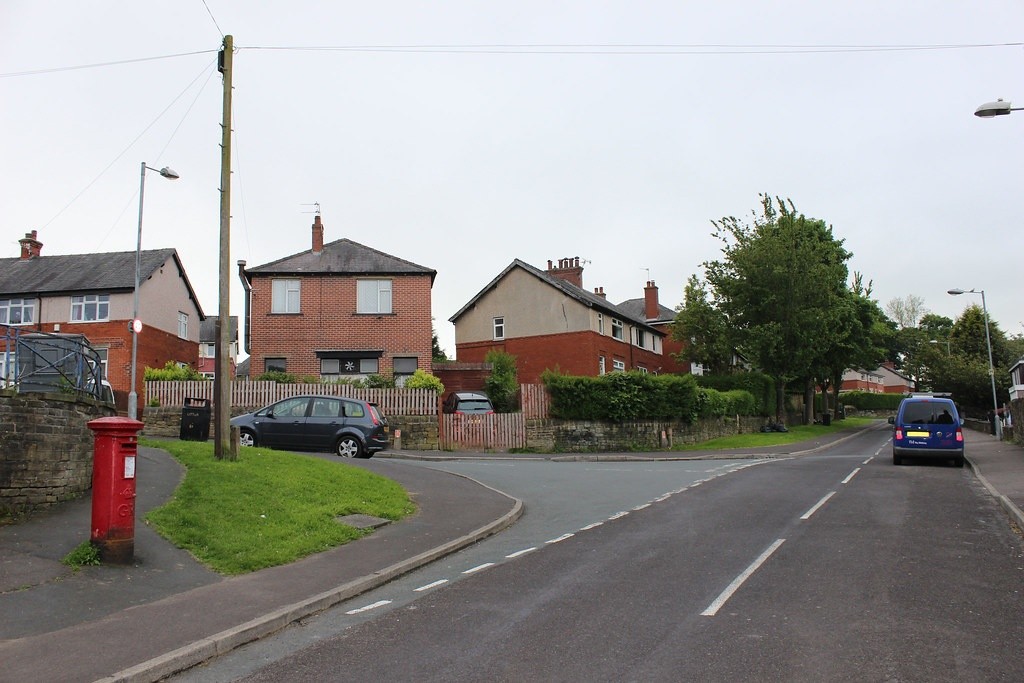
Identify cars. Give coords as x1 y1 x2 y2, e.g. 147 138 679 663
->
443 390 495 430
89 379 116 404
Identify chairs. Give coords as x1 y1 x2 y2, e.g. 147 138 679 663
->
314 404 334 416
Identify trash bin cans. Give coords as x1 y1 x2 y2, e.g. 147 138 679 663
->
17 331 92 396
180 397 211 442
822 413 831 426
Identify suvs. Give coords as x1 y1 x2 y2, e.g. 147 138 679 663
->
230 395 392 458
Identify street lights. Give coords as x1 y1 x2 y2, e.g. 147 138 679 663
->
948 289 1001 440
128 161 180 418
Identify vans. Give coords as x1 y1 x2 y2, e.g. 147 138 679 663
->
887 396 965 466
